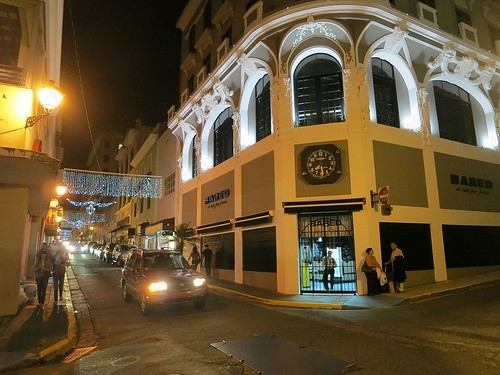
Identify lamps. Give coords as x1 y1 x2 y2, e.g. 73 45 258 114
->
25 80 67 129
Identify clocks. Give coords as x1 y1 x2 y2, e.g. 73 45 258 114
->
307 149 336 178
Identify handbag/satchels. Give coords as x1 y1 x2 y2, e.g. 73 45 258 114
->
362 259 373 273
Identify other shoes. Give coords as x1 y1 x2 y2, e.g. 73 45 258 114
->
36 302 45 311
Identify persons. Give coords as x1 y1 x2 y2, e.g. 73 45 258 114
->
323 251 337 291
33 242 51 310
202 245 213 278
385 242 407 292
50 250 68 305
188 247 200 271
365 248 383 296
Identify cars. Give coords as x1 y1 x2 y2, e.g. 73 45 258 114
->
112 243 139 268
62 238 116 264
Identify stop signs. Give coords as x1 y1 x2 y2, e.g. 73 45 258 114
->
378 185 389 206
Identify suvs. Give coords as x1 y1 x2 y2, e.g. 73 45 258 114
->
120 246 209 317
